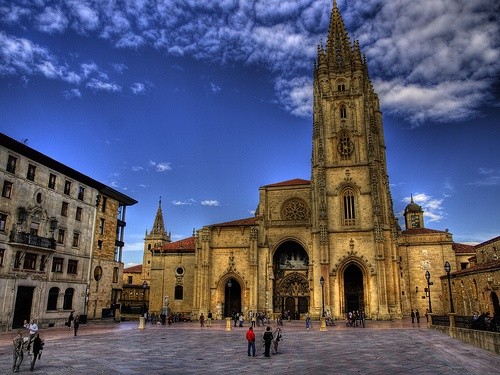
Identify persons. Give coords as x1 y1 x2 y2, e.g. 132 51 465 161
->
246 327 257 357
249 310 267 327
263 327 274 357
273 328 282 353
144 311 194 324
207 310 212 327
231 311 245 327
13 330 28 372
425 309 428 323
473 311 490 322
199 313 205 327
411 309 415 323
324 308 335 326
416 309 420 323
346 310 366 328
68 312 74 328
120 303 132 314
75 315 80 336
305 313 310 330
30 333 44 372
27 320 38 350
278 309 291 326
